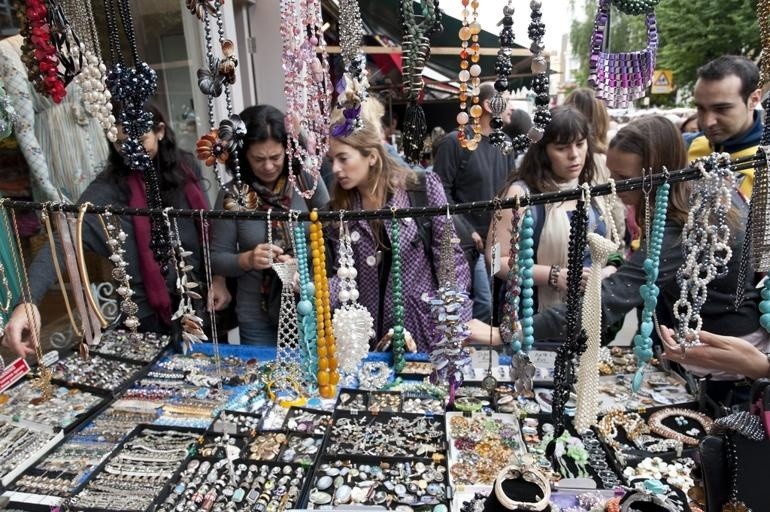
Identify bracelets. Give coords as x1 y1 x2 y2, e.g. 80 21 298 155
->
493 465 552 510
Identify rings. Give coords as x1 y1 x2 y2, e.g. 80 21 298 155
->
153 458 308 510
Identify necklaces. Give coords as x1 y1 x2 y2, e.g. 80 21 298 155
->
717 430 753 512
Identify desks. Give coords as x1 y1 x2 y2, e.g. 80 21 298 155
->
174 343 513 403
